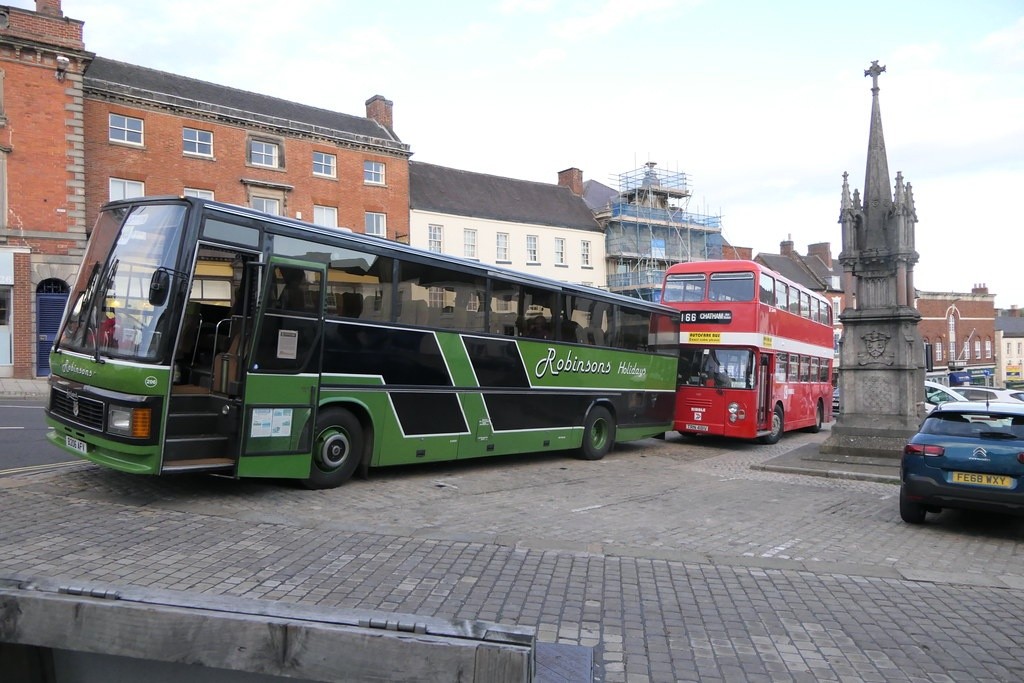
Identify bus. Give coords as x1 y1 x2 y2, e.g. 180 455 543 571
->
43 194 682 491
648 260 834 445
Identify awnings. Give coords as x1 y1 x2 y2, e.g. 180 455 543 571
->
951 371 971 384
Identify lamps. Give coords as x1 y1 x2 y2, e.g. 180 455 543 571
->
57 56 70 81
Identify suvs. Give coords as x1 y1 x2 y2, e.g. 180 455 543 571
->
900 401 1024 534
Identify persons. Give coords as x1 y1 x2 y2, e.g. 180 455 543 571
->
526 315 550 340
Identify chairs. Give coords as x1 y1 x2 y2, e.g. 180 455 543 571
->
282 285 605 347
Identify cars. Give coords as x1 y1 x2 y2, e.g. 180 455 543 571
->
833 387 840 409
923 381 1024 428
